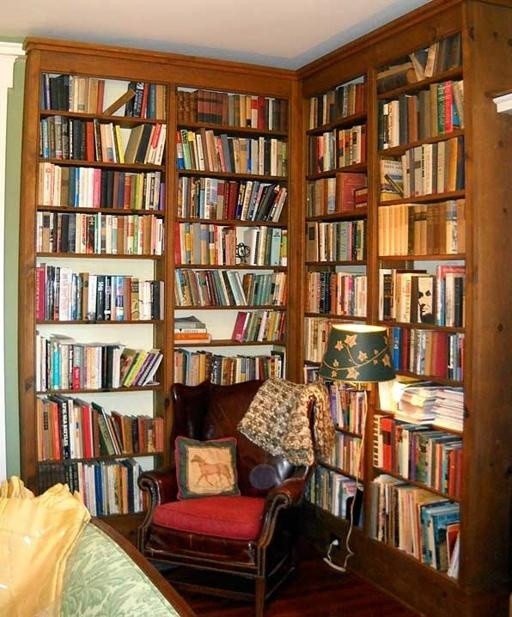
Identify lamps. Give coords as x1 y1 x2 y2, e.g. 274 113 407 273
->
316 323 396 574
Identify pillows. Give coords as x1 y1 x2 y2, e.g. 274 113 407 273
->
174 434 243 499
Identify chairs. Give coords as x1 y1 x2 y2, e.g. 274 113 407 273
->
135 379 316 617
0 477 193 617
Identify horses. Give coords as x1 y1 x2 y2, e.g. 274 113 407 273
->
191 453 232 488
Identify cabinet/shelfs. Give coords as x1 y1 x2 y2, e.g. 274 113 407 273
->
169 54 296 387
287 35 368 533
18 36 171 530
369 1 512 616
